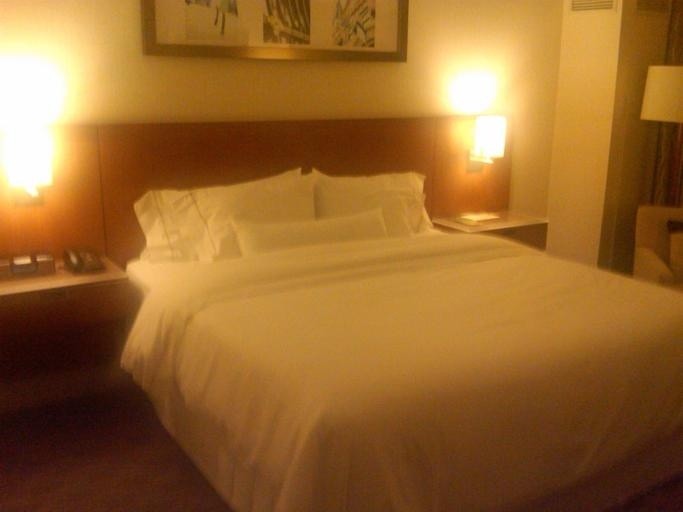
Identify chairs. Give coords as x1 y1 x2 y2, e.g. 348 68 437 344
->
633 205 683 292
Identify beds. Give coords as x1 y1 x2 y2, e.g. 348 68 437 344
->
98 115 683 512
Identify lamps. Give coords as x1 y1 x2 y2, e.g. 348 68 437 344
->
640 65 683 204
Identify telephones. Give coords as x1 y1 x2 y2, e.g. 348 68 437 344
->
63 246 105 274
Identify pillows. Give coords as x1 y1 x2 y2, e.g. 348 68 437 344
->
166 173 313 258
312 167 426 236
668 220 683 279
133 167 302 263
227 209 386 256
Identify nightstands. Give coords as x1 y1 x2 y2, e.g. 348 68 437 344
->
432 210 548 251
0 258 129 417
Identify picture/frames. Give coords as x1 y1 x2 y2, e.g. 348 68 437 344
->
141 0 408 63
